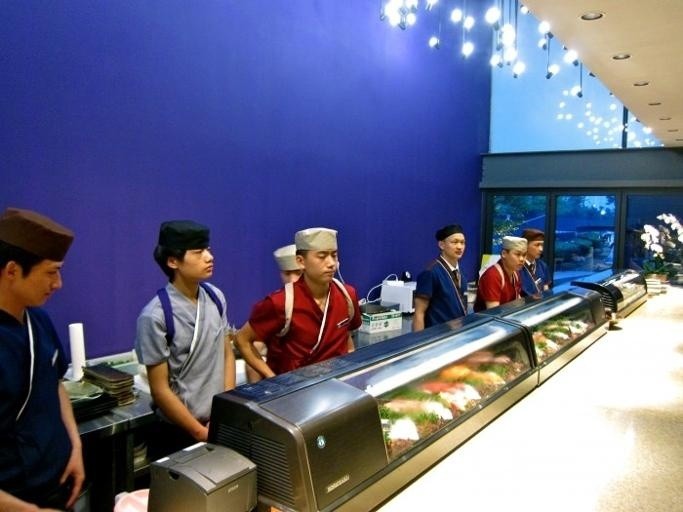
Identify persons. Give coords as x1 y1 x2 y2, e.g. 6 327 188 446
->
244 243 305 384
473 236 528 313
411 223 468 333
0 206 86 512
134 220 236 462
232 227 363 377
520 227 554 296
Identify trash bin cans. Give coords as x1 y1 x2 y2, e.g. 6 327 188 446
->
113 488 149 512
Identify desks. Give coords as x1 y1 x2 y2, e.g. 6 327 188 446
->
61 348 247 491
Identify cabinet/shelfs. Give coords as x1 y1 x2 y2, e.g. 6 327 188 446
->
481 284 608 384
210 314 538 510
571 268 649 320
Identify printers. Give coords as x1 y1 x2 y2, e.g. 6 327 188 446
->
148 441 257 512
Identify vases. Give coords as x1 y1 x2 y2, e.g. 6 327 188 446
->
649 270 668 293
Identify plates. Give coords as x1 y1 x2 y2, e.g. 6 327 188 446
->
82 364 136 407
73 396 119 422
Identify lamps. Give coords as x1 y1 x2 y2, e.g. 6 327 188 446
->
491 53 503 67
460 41 475 59
497 24 517 65
449 9 462 24
536 38 548 51
561 49 578 66
428 35 441 51
559 44 567 50
485 7 501 30
545 63 558 79
633 80 648 86
537 22 554 39
571 84 584 99
579 11 604 22
586 71 596 79
513 62 524 76
613 53 631 61
381 0 421 31
519 5 529 15
464 16 475 32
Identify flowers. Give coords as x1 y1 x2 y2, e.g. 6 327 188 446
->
637 212 683 258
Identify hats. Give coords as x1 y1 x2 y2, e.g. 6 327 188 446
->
523 228 544 241
1 207 74 260
159 219 211 250
274 244 304 270
295 228 338 249
502 236 527 251
435 223 464 242
